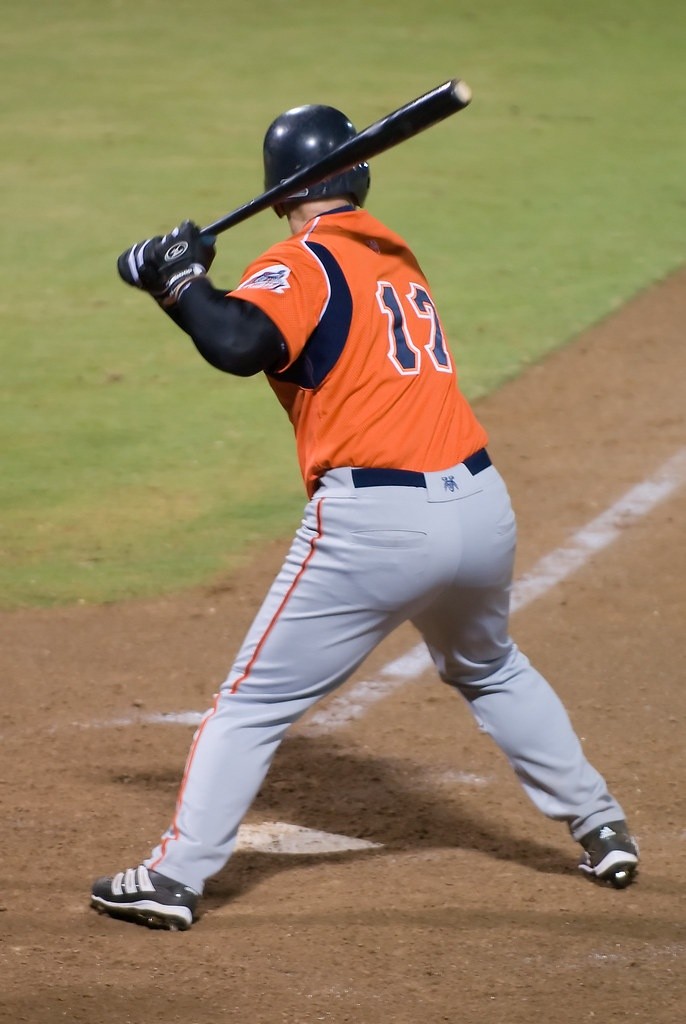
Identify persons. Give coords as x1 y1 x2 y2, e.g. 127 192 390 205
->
91 104 641 931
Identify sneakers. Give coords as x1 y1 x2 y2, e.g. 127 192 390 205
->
577 822 639 876
90 864 199 931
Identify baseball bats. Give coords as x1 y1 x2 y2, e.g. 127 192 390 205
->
198 77 474 240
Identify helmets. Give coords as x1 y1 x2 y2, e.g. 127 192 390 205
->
263 103 370 218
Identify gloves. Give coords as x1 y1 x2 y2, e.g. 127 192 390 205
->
118 237 171 304
149 219 216 301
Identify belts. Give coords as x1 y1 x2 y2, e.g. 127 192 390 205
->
313 447 492 491
